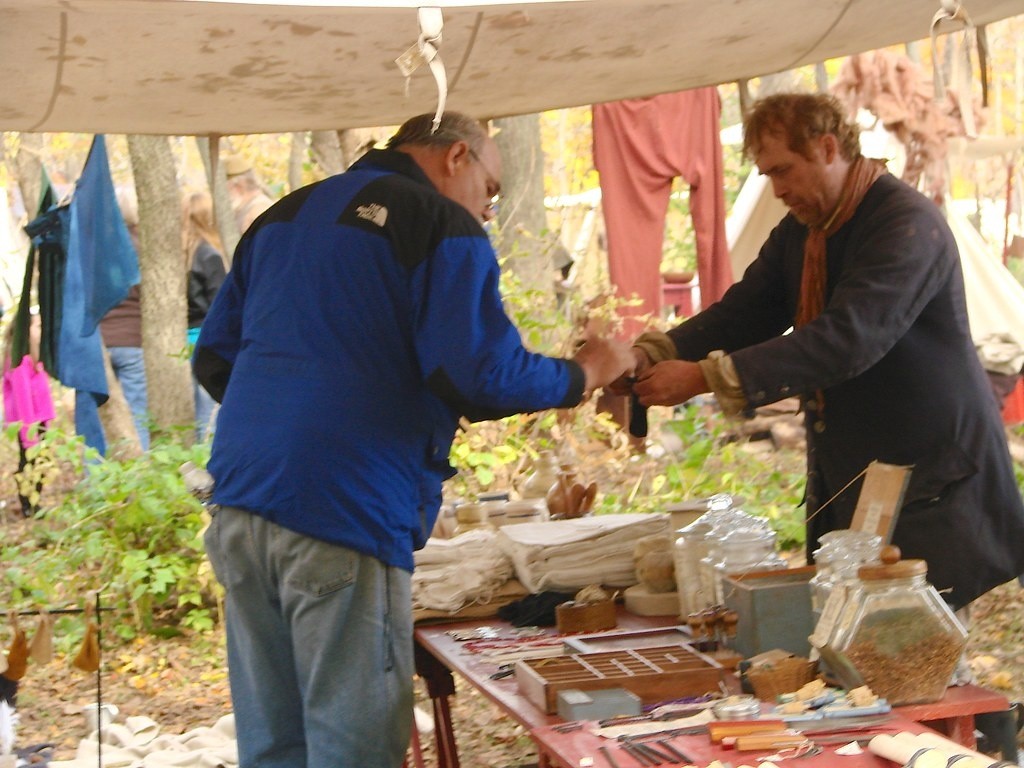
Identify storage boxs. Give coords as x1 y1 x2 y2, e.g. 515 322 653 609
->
515 644 725 714
719 459 913 656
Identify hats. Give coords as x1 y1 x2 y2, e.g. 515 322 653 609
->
223 159 252 181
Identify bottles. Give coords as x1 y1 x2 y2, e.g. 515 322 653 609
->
524 451 561 498
677 495 787 622
450 505 494 537
547 465 584 515
810 546 969 706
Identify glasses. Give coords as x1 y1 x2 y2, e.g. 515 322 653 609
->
469 147 505 211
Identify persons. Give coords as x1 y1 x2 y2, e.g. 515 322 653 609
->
191 113 639 767
603 92 1024 612
0 160 274 518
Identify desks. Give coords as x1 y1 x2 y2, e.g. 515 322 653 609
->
416 619 1012 768
